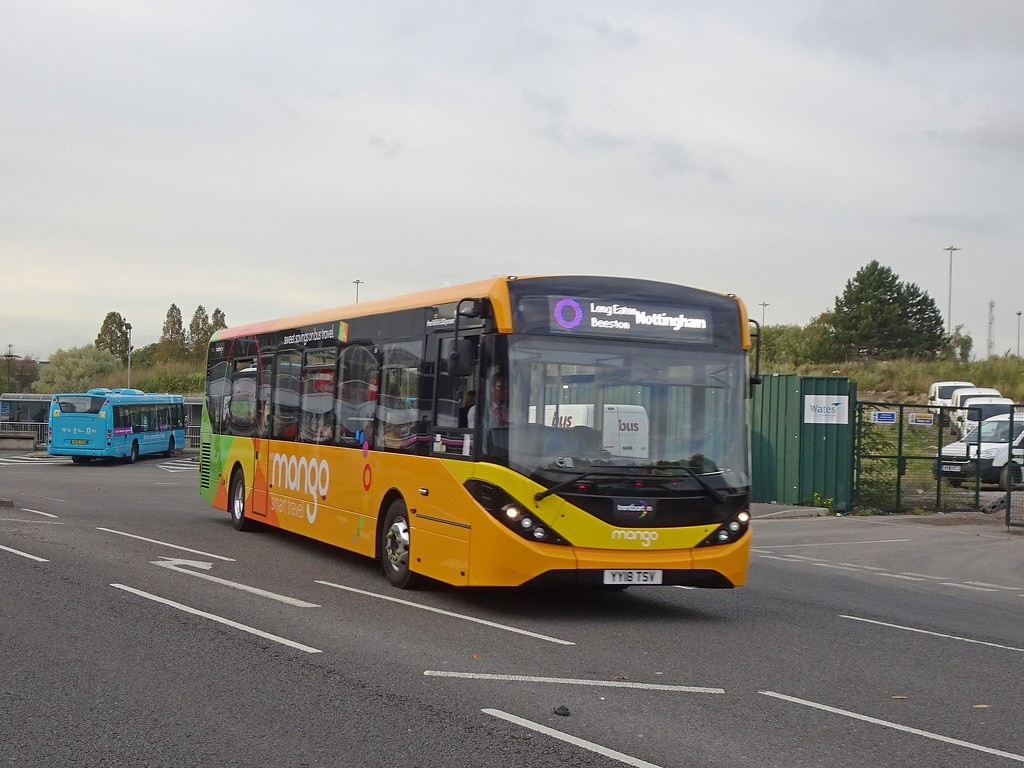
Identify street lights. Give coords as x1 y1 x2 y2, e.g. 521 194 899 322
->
125 322 132 389
1016 311 1022 358
943 244 961 338
759 302 770 327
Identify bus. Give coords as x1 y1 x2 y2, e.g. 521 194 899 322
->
197 275 761 592
47 387 186 464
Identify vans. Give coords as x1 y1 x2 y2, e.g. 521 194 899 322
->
927 381 1024 492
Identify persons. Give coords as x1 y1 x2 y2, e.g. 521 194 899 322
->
467 369 527 448
11 408 23 422
31 407 46 423
